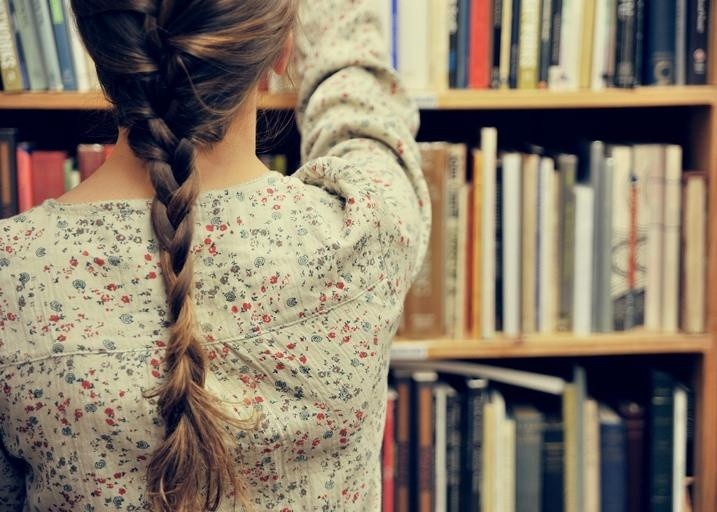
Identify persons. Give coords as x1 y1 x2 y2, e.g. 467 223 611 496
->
0 0 432 511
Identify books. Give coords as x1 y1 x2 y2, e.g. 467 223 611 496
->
377 358 696 512
252 0 713 93
1 1 121 217
260 127 710 340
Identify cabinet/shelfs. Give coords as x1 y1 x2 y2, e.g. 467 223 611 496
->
0 0 717 512
